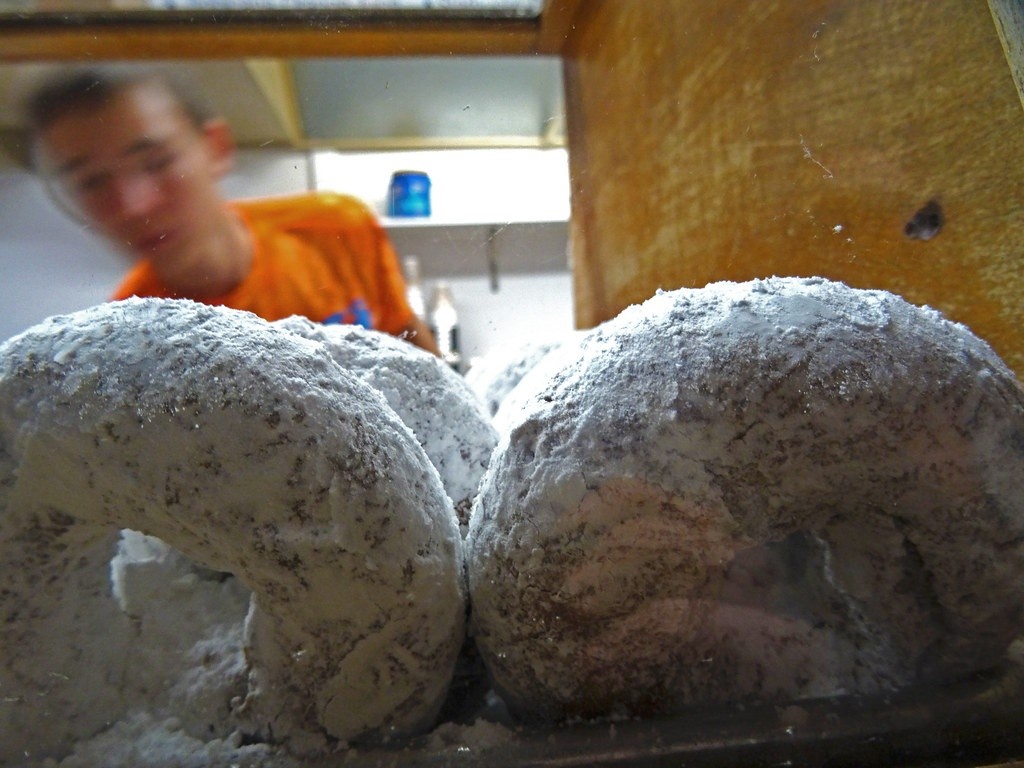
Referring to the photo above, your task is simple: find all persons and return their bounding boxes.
[18,58,443,360]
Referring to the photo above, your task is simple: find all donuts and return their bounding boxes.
[0,275,1024,768]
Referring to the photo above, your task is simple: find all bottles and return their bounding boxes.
[432,281,462,366]
[405,255,427,326]
